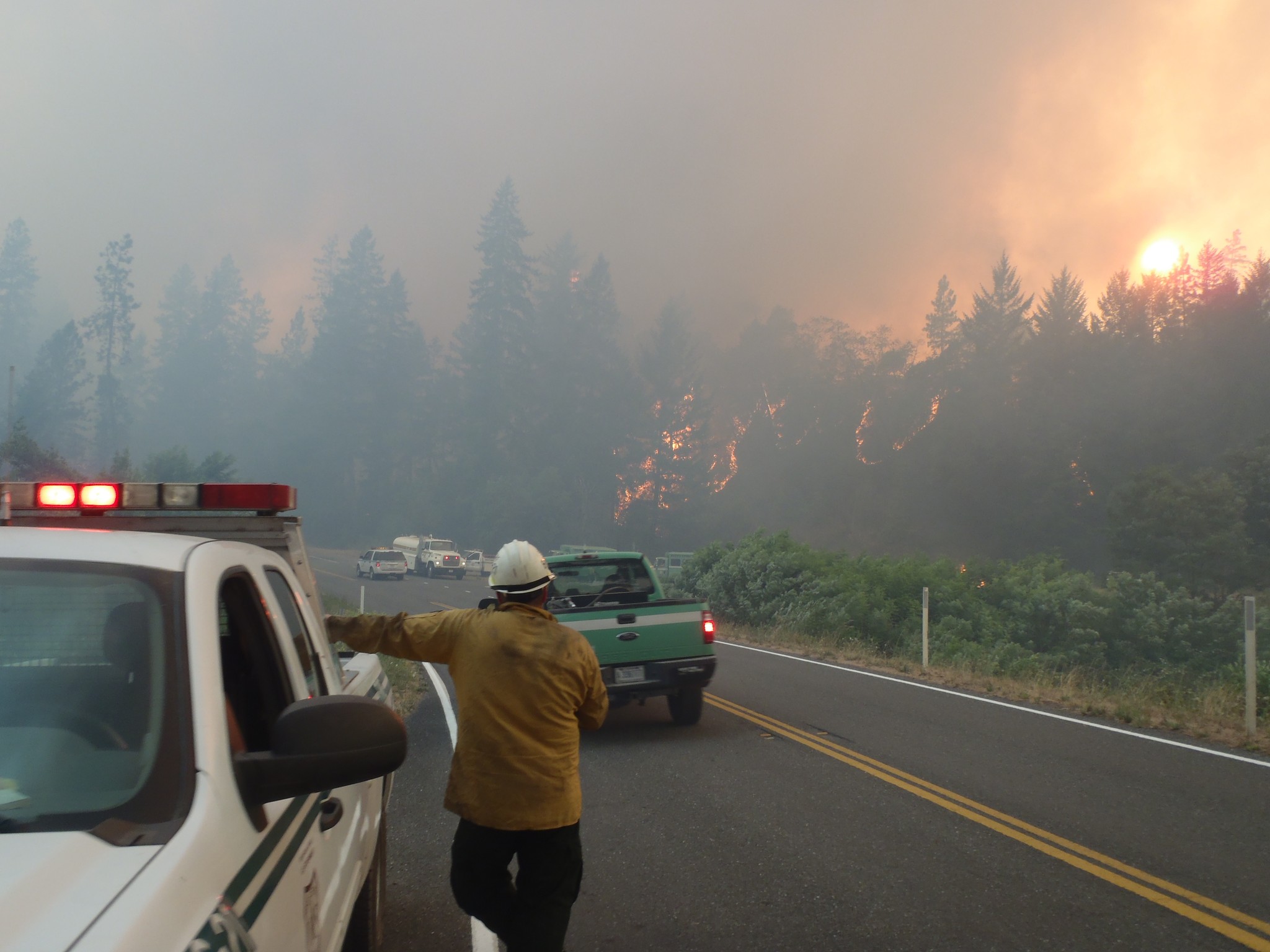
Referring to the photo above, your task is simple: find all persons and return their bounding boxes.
[324,540,609,952]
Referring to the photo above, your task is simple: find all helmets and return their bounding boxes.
[487,539,557,594]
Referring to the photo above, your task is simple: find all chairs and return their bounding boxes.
[600,574,626,594]
[103,603,148,733]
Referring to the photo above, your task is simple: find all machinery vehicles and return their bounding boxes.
[392,536,467,581]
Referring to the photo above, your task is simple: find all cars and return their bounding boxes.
[356,549,407,580]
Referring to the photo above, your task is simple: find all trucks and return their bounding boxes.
[654,551,694,584]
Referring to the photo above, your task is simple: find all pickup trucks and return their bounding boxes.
[479,551,716,725]
[461,549,496,577]
[0,524,410,952]
